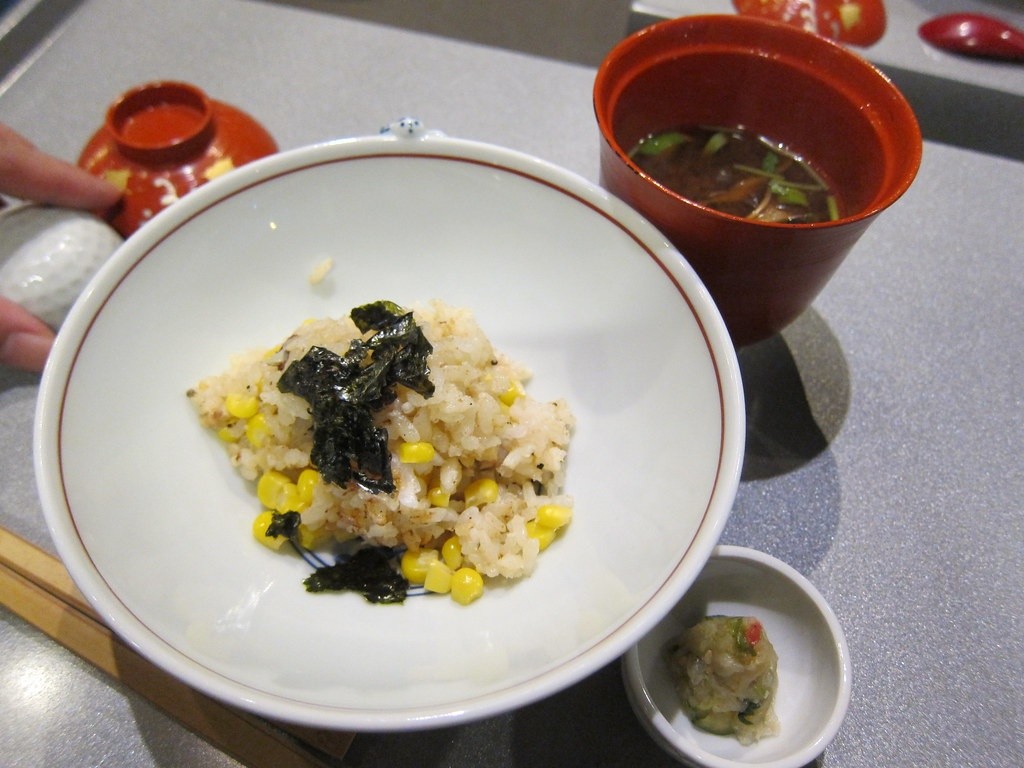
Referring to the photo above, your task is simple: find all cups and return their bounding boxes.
[593,15,924,350]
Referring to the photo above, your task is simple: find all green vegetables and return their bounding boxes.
[636,128,840,222]
[274,297,437,604]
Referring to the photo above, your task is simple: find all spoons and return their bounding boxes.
[915,14,1024,64]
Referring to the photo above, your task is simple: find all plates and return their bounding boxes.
[628,0,1024,96]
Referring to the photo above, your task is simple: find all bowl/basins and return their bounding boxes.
[621,545,852,768]
[31,114,746,732]
[77,80,279,242]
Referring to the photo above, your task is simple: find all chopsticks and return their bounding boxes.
[0,526,358,768]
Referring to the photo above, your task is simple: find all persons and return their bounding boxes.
[0,125,125,376]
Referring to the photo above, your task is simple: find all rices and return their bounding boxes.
[184,257,573,579]
